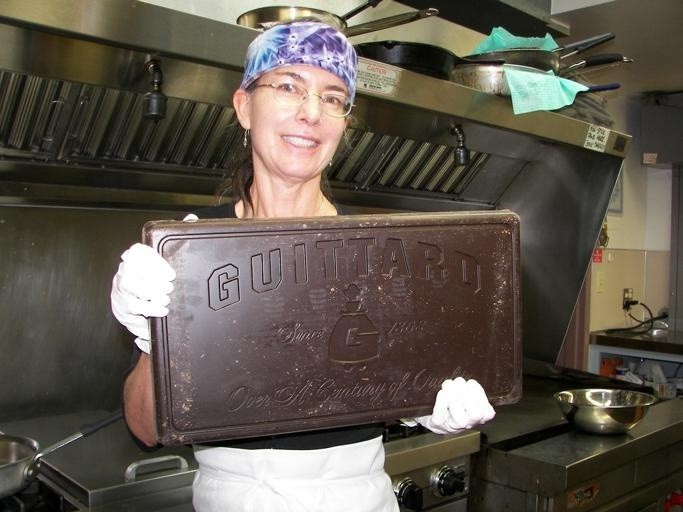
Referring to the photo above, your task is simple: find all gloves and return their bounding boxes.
[413,377,496,435]
[110,214,199,353]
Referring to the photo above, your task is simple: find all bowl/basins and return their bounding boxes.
[553,388,656,435]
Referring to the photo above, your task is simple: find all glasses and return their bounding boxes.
[256,82,357,118]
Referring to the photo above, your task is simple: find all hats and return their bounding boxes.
[242,21,358,106]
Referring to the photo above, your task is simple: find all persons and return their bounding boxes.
[112,27,497,511]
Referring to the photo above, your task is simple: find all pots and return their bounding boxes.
[238,0,629,96]
[0,410,127,496]
